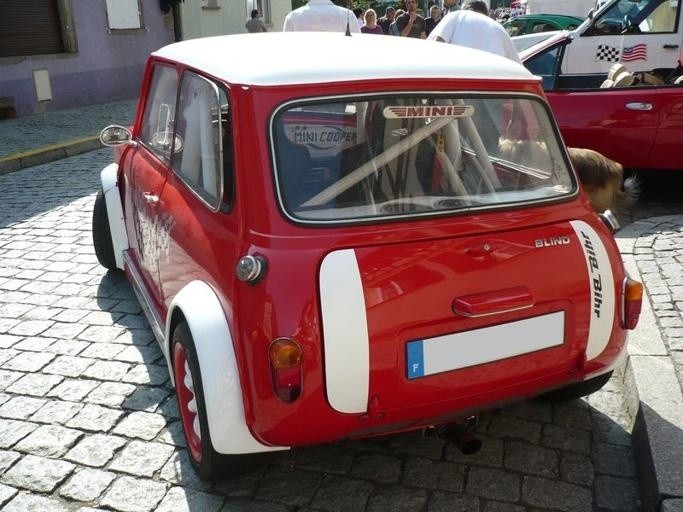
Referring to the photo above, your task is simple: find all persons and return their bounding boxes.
[282,0,520,66]
[245,10,267,33]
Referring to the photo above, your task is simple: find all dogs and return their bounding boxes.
[496,135,640,217]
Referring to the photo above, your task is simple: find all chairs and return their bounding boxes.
[337,98,447,206]
[597,63,634,89]
[197,102,311,205]
